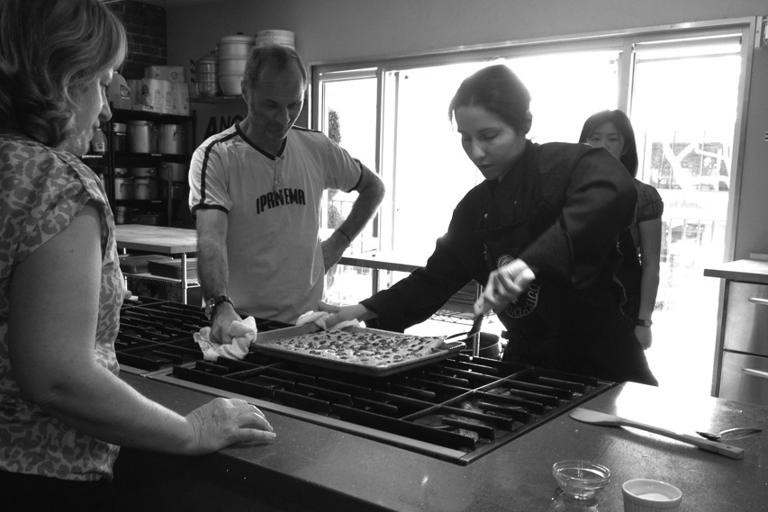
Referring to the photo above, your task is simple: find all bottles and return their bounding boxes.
[91,127,108,152]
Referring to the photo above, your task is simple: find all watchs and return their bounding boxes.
[636,318,652,327]
[202,295,231,321]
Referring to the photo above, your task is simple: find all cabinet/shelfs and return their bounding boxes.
[104,100,196,226]
[703,255,768,405]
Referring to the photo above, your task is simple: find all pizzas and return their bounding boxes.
[267,327,446,370]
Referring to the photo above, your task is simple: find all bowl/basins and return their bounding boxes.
[551,458,611,500]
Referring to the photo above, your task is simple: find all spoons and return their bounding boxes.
[696,426,763,440]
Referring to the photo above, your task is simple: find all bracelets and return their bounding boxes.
[334,226,352,243]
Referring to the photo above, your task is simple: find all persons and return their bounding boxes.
[317,63,657,386]
[577,108,666,353]
[2,0,278,512]
[185,43,386,348]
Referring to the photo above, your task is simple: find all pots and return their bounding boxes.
[189,30,296,97]
[113,118,187,224]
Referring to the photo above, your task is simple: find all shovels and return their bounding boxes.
[569,407,745,460]
[441,269,536,346]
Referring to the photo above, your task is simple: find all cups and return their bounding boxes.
[621,478,683,512]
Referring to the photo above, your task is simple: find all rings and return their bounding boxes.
[640,344,646,349]
